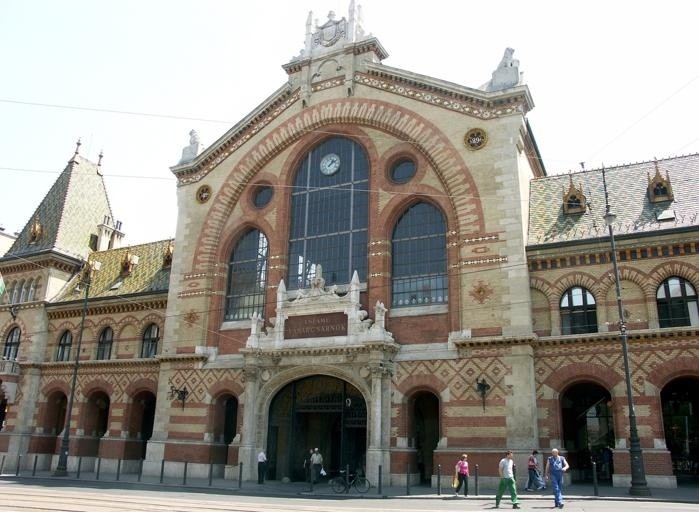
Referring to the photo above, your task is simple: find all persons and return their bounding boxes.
[303,448,323,484]
[526,450,545,491]
[257,449,267,484]
[496,450,521,509]
[455,454,469,497]
[545,448,569,509]
[416,446,424,473]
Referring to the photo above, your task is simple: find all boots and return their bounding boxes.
[513,504,521,509]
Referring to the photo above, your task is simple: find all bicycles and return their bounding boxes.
[331,469,371,494]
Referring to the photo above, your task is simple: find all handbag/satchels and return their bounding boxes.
[451,478,459,489]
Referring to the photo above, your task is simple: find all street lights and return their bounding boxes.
[52,257,92,476]
[601,169,651,496]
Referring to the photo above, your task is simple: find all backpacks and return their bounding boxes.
[533,470,547,490]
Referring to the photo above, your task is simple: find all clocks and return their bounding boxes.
[320,153,340,175]
